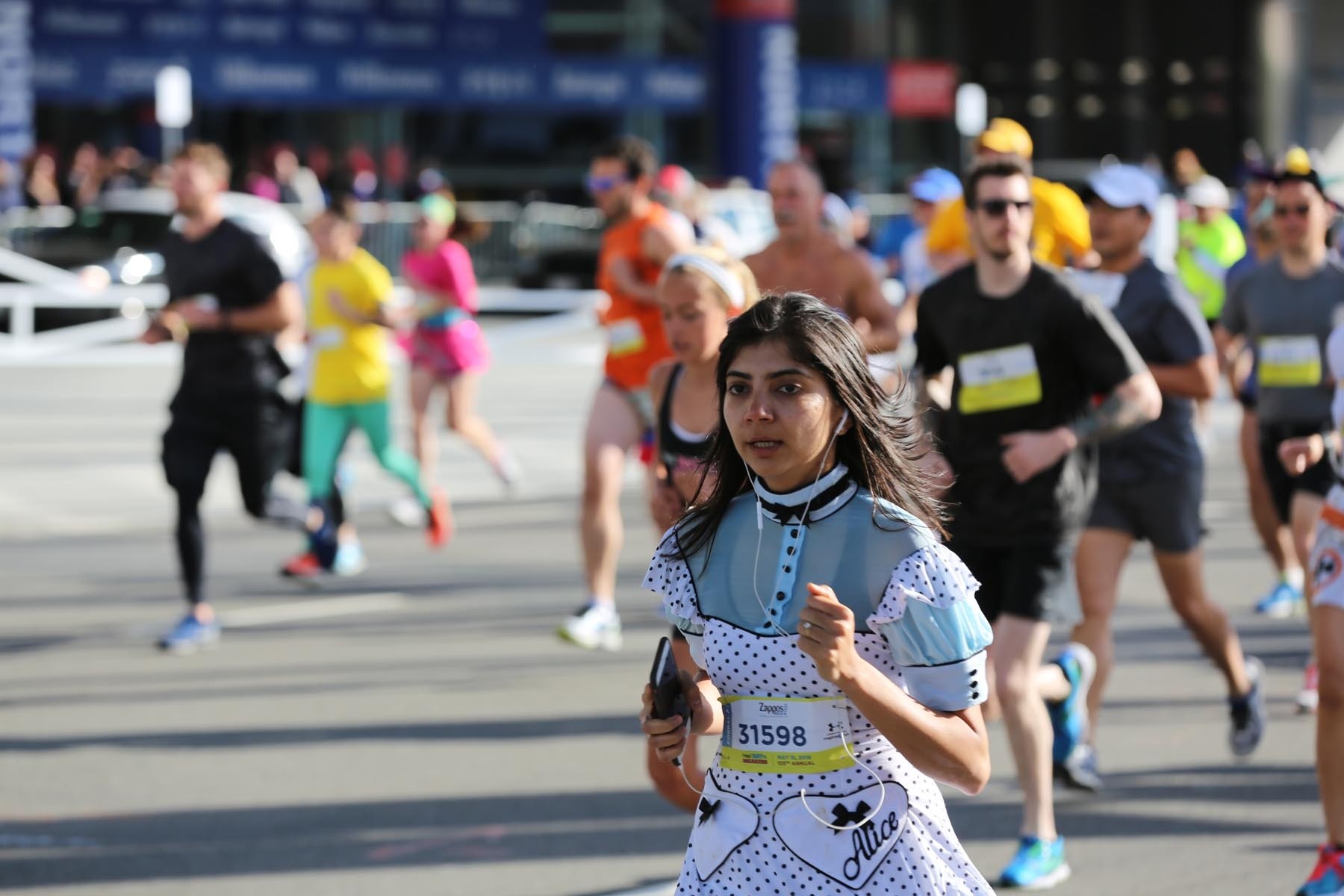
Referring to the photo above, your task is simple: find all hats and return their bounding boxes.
[1077,163,1160,218]
[910,167,963,205]
[978,117,1033,160]
[1184,174,1228,209]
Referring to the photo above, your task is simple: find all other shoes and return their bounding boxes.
[333,539,369,576]
[557,604,623,651]
[425,488,453,543]
[156,616,220,653]
[284,551,329,578]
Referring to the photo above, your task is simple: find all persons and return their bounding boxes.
[1062,171,1264,790]
[0,141,519,661]
[900,117,1246,430]
[548,139,900,654]
[913,162,1161,891]
[1219,142,1344,896]
[640,295,999,896]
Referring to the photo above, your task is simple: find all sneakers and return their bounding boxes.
[1229,657,1267,752]
[1293,660,1320,712]
[999,832,1070,888]
[1294,840,1344,896]
[1043,642,1097,763]
[1256,582,1310,619]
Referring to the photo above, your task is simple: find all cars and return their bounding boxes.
[0,183,320,358]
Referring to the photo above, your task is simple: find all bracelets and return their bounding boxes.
[1069,415,1099,448]
[1318,430,1333,453]
[219,309,231,329]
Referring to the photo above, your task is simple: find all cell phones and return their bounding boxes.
[649,636,694,767]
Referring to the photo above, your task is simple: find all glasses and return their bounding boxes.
[587,173,630,191]
[972,198,1033,216]
[1270,204,1327,219]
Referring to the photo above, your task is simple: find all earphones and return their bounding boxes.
[833,406,850,437]
